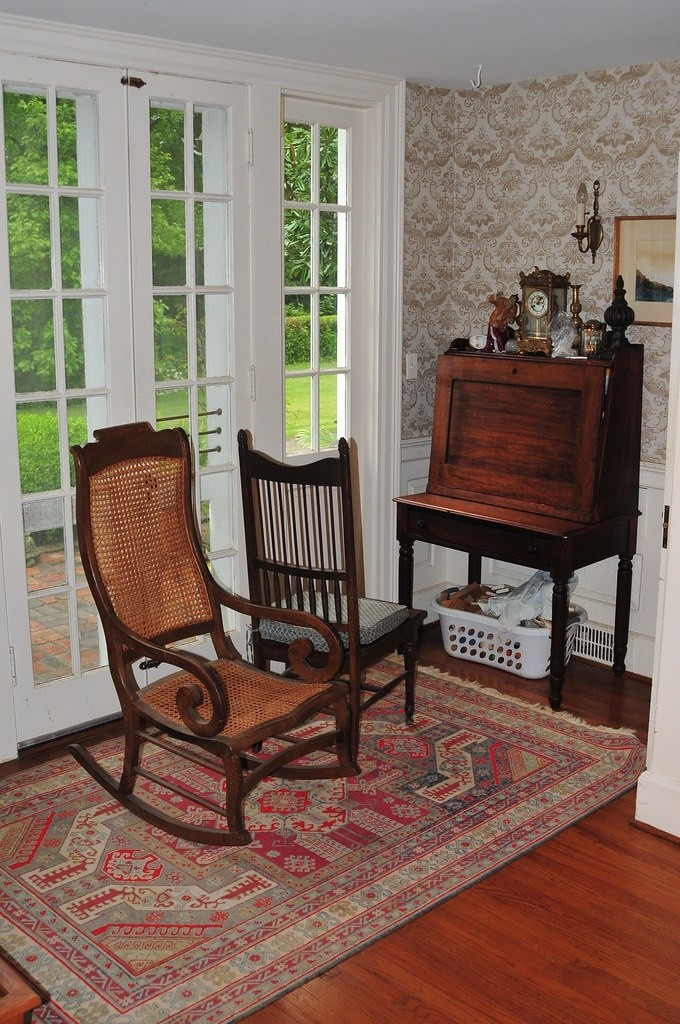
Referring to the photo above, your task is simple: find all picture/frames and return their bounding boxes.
[612,214,676,329]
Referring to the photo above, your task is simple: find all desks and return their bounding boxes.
[388,493,642,710]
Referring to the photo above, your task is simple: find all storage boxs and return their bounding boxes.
[431,588,588,679]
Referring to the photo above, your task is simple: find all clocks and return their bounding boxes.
[517,266,571,355]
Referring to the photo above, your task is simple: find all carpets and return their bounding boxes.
[0,650,648,1024]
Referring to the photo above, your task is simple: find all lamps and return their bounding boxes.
[567,180,602,265]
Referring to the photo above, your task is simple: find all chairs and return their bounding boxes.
[231,427,429,757]
[66,422,361,849]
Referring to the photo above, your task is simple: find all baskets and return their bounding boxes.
[431,584,587,678]
